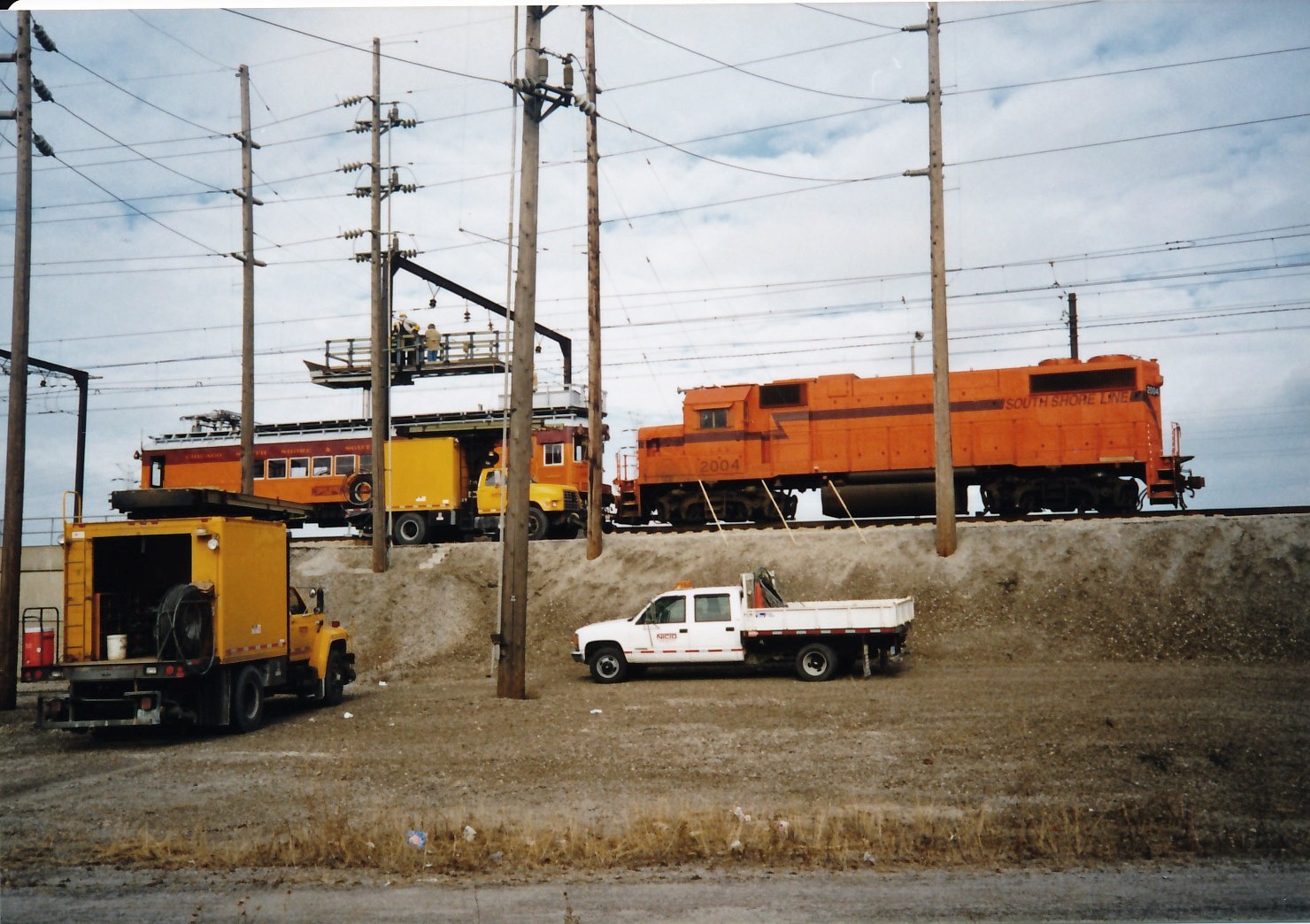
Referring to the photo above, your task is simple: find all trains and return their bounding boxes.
[613,352,1205,531]
[133,402,611,541]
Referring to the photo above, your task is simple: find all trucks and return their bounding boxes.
[380,434,582,544]
[19,485,358,734]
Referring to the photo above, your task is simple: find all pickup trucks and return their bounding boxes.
[573,571,916,682]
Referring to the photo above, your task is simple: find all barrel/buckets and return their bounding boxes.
[25,627,54,679]
[107,634,128,661]
